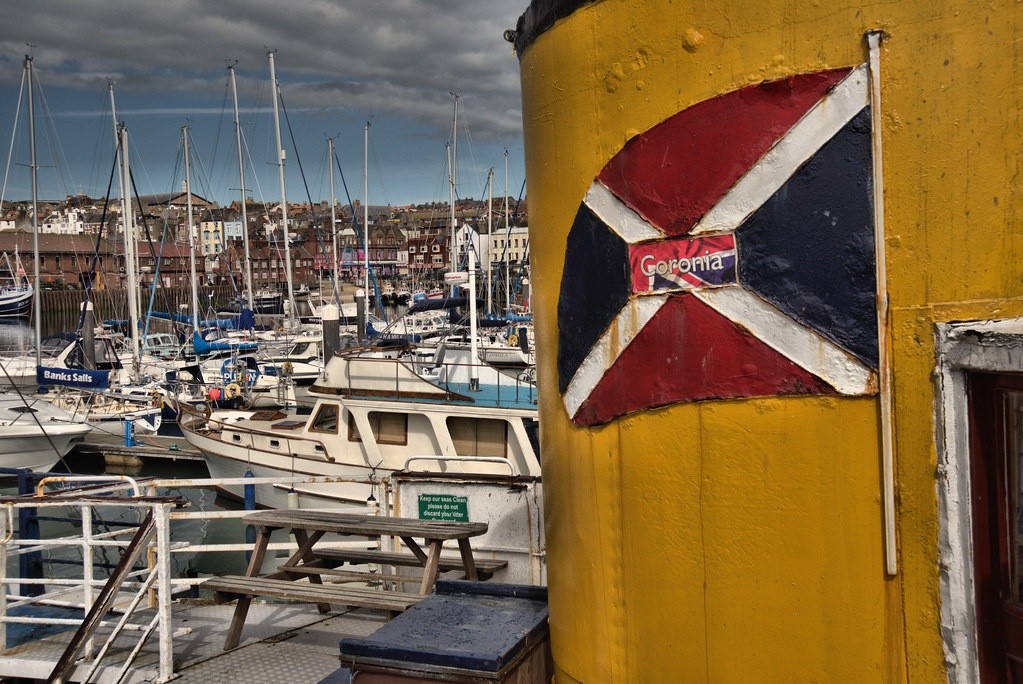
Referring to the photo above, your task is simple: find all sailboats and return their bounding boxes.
[0,53,541,435]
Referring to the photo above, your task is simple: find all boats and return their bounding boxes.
[173,247,541,516]
[1,391,92,481]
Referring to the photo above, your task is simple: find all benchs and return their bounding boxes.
[200,574,430,611]
[311,548,510,573]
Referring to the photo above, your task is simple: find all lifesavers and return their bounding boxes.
[225,383,240,398]
[506,335,518,346]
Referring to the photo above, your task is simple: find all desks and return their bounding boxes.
[224,510,493,650]
